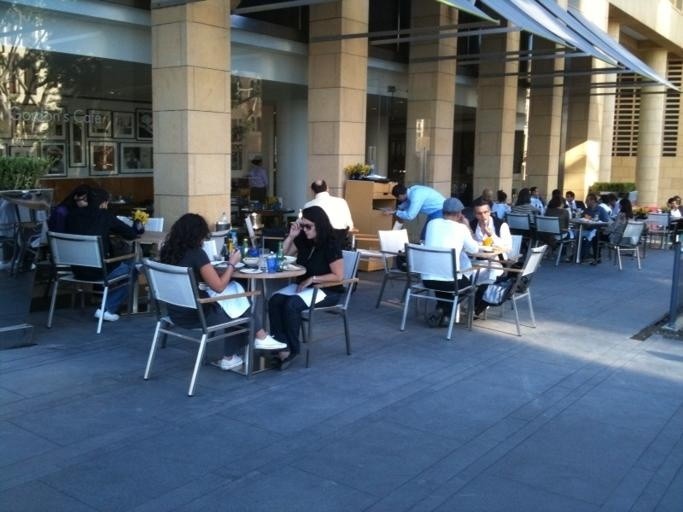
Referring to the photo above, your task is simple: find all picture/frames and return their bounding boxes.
[0,102,153,177]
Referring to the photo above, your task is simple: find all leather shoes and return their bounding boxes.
[281,352,299,370]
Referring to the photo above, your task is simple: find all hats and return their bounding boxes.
[443,197,465,212]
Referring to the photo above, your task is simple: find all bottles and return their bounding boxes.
[219,232,284,272]
[220,212,227,224]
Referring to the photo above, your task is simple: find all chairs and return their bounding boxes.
[376,228,420,308]
[400,243,480,340]
[491,206,683,271]
[467,244,548,336]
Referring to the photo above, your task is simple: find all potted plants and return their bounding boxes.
[345,162,370,179]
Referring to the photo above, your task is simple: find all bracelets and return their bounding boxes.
[229,264,236,272]
[313,275,317,284]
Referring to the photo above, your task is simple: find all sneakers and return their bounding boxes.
[221,354,243,370]
[254,335,287,349]
[95,308,120,321]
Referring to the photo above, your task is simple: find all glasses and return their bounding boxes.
[299,224,314,229]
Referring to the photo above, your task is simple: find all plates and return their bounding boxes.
[240,269,263,274]
[210,261,245,268]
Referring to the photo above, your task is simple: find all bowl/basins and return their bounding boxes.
[268,255,298,264]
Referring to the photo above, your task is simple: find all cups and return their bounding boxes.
[483,237,492,246]
[595,215,599,222]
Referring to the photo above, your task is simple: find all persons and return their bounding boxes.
[268,206,346,370]
[469,198,513,321]
[385,183,444,245]
[248,155,268,203]
[65,190,138,322]
[159,213,287,372]
[472,184,683,264]
[426,198,479,327]
[47,184,95,302]
[310,180,354,249]
[231,178,252,226]
